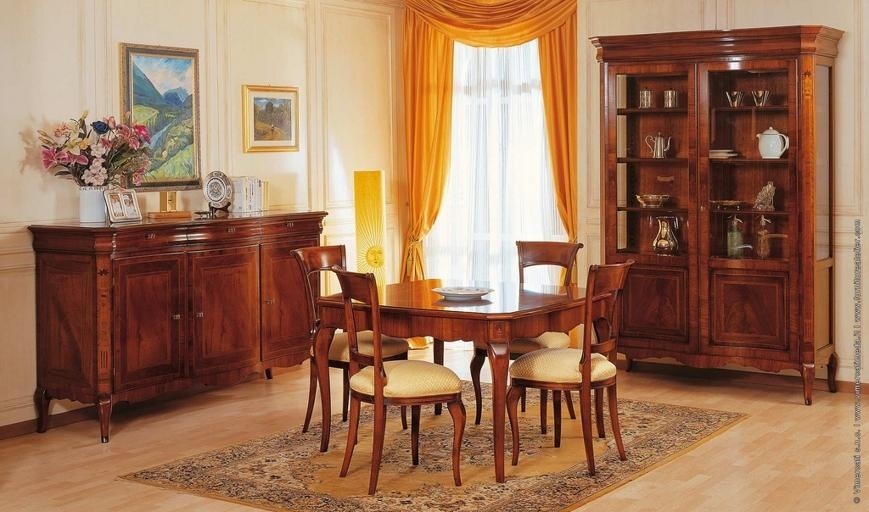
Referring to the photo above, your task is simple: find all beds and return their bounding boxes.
[306,274,615,484]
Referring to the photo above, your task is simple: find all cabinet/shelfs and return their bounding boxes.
[582,22,848,408]
[23,213,262,445]
[255,208,333,383]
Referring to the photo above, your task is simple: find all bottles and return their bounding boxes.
[756,215,773,257]
[727,218,745,259]
[652,216,679,256]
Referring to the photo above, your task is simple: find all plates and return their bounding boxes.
[202,171,233,209]
[709,149,738,159]
[432,287,493,302]
[710,200,748,207]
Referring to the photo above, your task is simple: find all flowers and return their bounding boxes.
[32,103,155,193]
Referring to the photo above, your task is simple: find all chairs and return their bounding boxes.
[464,234,585,424]
[285,239,412,456]
[325,259,466,498]
[497,255,644,480]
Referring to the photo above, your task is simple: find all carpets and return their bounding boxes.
[117,377,752,512]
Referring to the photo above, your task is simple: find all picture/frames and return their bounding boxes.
[101,184,144,225]
[113,38,209,195]
[239,81,301,153]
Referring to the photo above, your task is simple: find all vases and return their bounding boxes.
[73,181,115,225]
[652,214,681,258]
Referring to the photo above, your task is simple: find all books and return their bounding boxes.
[229,175,271,213]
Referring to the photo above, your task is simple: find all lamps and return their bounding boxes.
[352,166,389,307]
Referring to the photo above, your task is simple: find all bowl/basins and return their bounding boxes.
[635,195,670,209]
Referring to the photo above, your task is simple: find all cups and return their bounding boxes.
[663,89,678,109]
[725,91,744,108]
[639,89,656,109]
[752,90,770,107]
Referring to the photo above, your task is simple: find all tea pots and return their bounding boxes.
[756,127,789,160]
[645,132,672,159]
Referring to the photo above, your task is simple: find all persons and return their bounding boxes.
[122,195,138,217]
[110,197,123,217]
